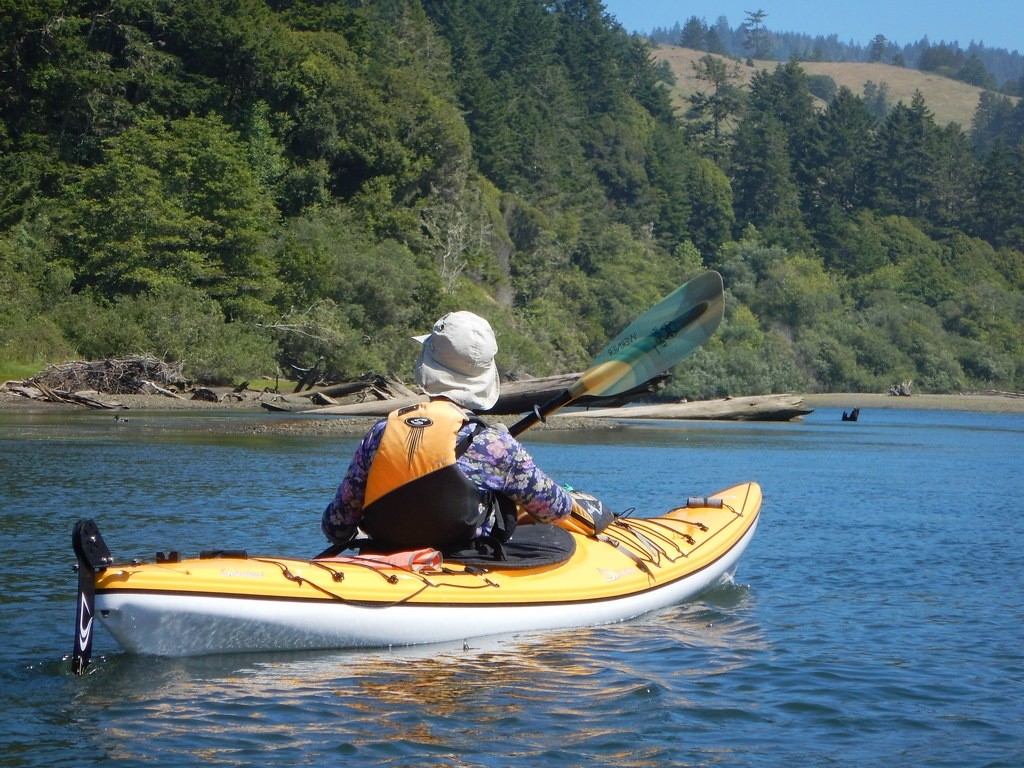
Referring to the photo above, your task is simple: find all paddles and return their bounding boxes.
[308,261,732,566]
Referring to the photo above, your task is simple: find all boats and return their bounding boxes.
[71,481,763,677]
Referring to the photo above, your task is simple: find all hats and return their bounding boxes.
[415,311,500,411]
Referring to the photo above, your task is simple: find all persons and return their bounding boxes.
[322,310,610,551]
